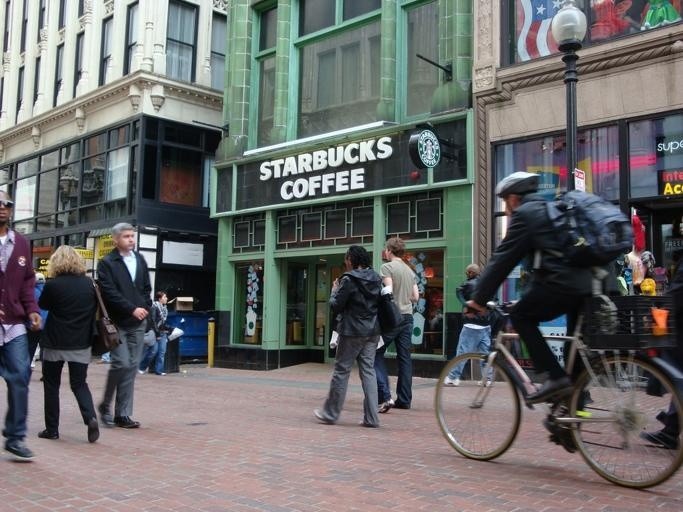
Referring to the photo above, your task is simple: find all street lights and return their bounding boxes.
[547,0,588,333]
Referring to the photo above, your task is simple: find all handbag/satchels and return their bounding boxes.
[379,294,403,332]
[89,277,122,357]
[142,329,156,346]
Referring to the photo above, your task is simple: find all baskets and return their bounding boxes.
[583,291,680,352]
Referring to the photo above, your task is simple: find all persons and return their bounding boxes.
[313,244,386,429]
[373,237,417,410]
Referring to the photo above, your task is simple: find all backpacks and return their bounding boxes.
[521,189,634,270]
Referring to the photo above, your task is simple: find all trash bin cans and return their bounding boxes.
[149,325,181,373]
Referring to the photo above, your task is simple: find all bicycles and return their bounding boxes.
[432,285,682,492]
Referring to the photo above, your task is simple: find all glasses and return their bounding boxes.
[0,200,14,209]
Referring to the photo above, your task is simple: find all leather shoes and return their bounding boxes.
[523,375,575,405]
[99,403,114,425]
[640,429,680,451]
[114,416,141,428]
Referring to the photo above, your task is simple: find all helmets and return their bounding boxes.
[495,170,540,199]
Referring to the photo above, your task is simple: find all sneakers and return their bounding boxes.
[478,379,495,388]
[37,428,59,439]
[443,376,460,387]
[313,409,333,425]
[3,440,34,461]
[379,400,412,414]
[88,416,100,443]
